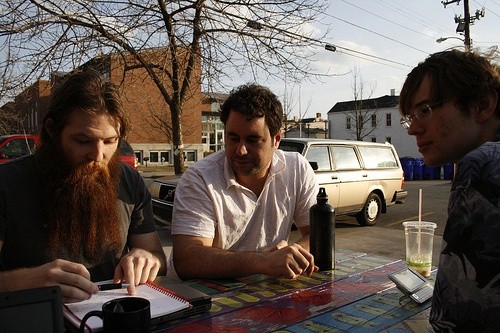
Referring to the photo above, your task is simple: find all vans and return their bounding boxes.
[0,134,140,174]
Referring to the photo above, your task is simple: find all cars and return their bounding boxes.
[153,137,411,241]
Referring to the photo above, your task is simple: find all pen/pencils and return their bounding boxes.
[98,283,130,291]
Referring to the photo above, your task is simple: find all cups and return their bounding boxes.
[79,297,151,333]
[402,221,437,277]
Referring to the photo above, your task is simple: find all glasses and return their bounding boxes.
[400,103,445,130]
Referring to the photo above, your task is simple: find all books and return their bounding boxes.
[61,278,193,333]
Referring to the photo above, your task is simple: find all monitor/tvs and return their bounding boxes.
[389,265,429,295]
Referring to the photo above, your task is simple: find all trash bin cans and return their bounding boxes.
[399,156,453,181]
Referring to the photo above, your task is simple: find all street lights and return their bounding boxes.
[436,35,472,55]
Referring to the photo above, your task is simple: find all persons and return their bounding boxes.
[0,72,168,305]
[399,49,500,333]
[170,84,320,282]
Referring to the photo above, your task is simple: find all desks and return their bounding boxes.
[152,249,439,333]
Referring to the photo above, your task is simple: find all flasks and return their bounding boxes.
[309,187,335,271]
